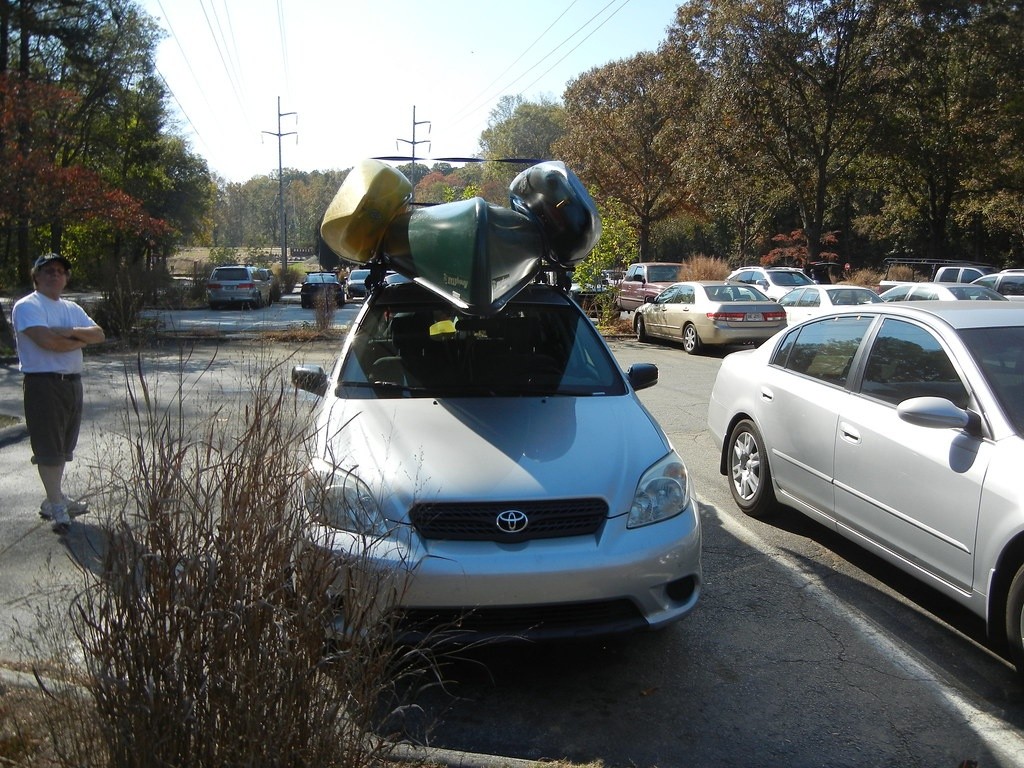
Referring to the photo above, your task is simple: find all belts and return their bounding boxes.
[37,372,82,381]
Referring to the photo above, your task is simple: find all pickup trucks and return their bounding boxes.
[877,266,999,293]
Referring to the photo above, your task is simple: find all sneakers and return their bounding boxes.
[51,503,71,533]
[37,494,87,517]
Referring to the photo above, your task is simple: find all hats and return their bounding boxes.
[34,251,72,270]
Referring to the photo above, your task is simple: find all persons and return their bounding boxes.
[12,253,106,529]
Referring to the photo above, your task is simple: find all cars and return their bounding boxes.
[707,300,1024,691]
[612,262,690,311]
[772,284,886,327]
[300,272,345,309]
[207,264,275,309]
[631,280,788,356]
[861,282,1011,301]
[563,268,627,318]
[344,270,372,299]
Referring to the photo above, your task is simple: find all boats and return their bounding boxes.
[319,155,413,261]
[510,160,601,269]
[390,194,546,316]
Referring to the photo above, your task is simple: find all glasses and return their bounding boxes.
[42,267,68,276]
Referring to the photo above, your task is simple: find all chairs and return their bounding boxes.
[369,312,561,374]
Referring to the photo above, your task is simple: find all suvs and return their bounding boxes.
[290,267,704,648]
[714,265,819,304]
[964,269,1024,302]
